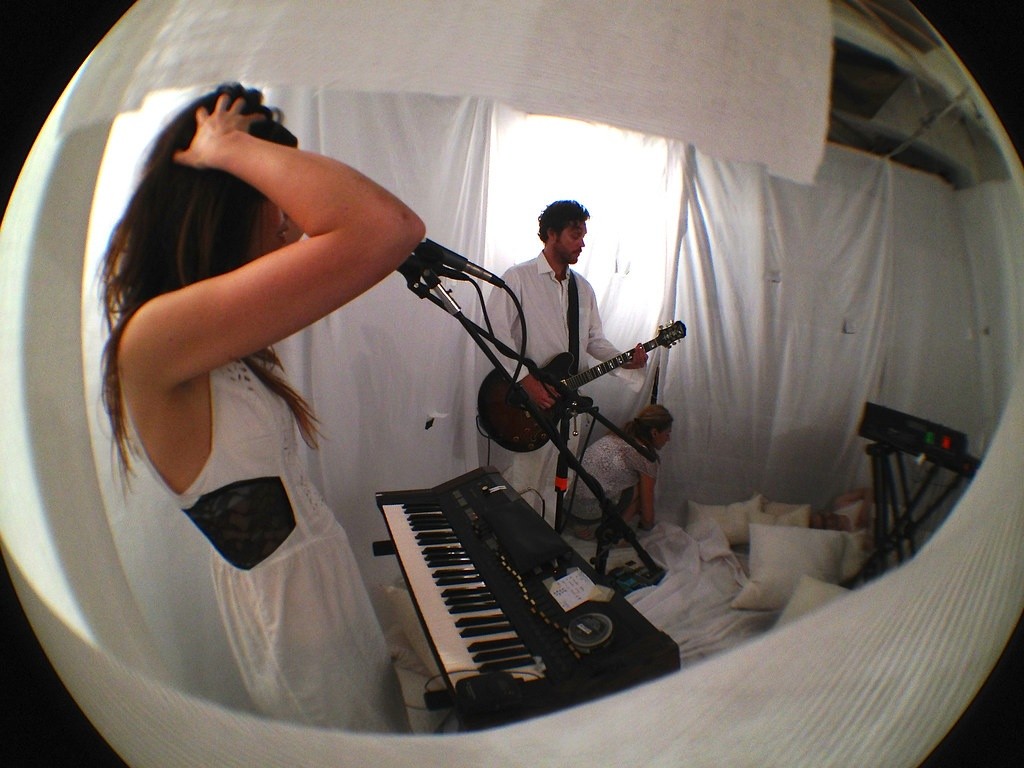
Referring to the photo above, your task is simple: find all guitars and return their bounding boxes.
[475,314,686,452]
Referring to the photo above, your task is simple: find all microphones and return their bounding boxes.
[414,237,505,289]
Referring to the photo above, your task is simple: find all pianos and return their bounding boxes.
[373,465,686,736]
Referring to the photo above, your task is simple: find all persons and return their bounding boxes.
[481,200,648,531]
[807,486,908,560]
[561,405,674,547]
[103,82,426,735]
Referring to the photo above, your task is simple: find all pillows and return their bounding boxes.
[748,503,811,528]
[769,576,854,634]
[728,523,844,611]
[687,494,763,544]
